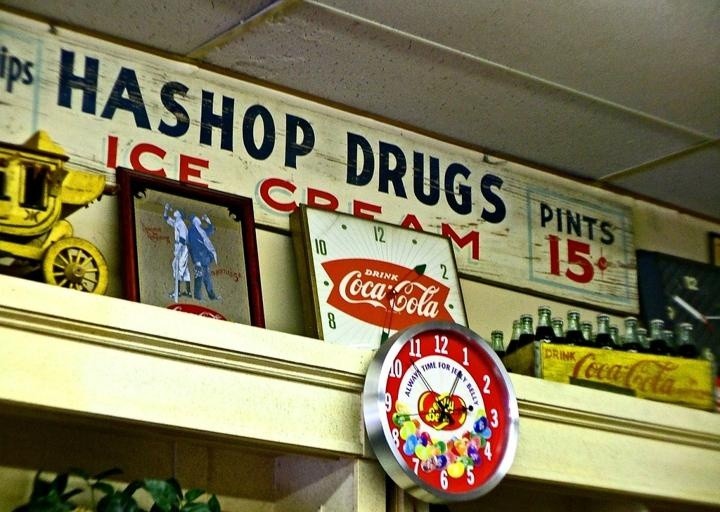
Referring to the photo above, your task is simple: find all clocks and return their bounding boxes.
[639,245,718,356]
[365,322,521,503]
[289,198,477,356]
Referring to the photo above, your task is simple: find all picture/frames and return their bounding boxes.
[115,164,270,332]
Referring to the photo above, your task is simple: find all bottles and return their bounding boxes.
[490,308,700,360]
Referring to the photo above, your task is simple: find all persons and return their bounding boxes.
[164,203,190,298]
[187,213,218,299]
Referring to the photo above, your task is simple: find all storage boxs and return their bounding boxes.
[500,337,717,412]
